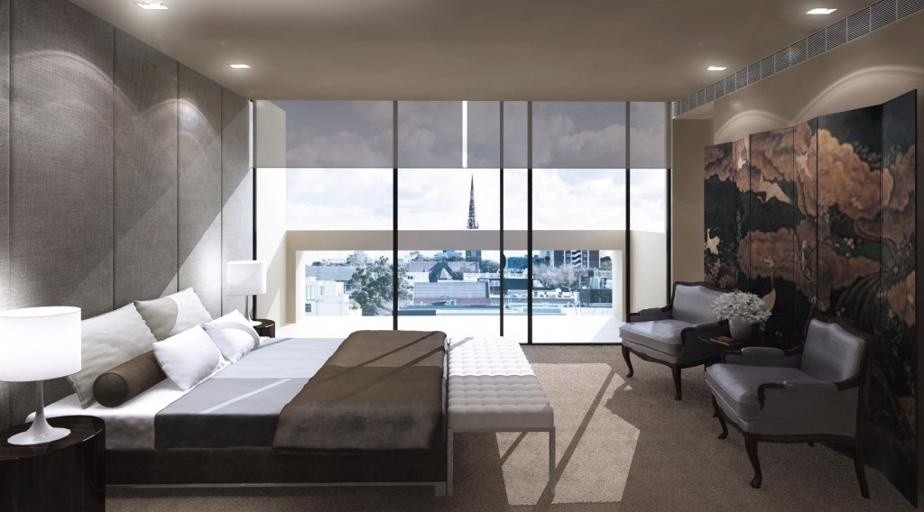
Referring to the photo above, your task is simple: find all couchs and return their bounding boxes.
[702,309,873,499]
[619,280,735,401]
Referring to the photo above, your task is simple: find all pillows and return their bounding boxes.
[153,323,221,391]
[204,307,261,365]
[135,287,214,341]
[65,302,157,410]
[93,351,167,408]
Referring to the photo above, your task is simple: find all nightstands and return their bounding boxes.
[0,416,106,512]
[250,318,275,338]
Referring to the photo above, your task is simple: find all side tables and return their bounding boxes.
[698,322,805,418]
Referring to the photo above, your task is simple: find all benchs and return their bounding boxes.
[447,333,556,499]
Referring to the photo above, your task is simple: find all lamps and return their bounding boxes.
[224,259,268,321]
[0,305,83,447]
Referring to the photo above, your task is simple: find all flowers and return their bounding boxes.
[710,284,773,332]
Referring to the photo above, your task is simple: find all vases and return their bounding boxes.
[728,316,759,341]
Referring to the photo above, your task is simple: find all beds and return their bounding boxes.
[9,331,447,498]
